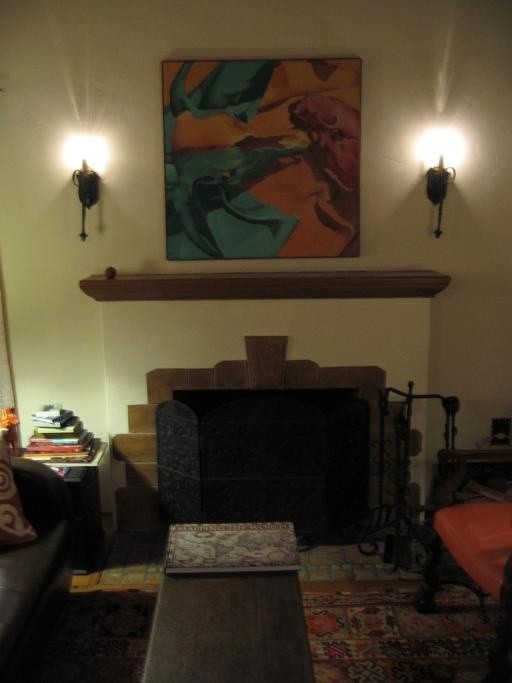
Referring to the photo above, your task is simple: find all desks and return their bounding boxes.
[143,520,318,683]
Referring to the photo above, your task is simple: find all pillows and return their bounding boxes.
[1,446,39,547]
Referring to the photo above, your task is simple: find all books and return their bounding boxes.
[163,520,301,574]
[21,403,108,468]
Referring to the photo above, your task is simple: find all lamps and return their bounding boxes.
[57,127,113,242]
[411,124,470,241]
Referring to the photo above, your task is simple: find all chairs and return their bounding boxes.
[419,499,511,621]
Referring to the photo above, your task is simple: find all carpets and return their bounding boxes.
[16,565,510,680]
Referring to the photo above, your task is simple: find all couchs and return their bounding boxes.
[2,453,86,682]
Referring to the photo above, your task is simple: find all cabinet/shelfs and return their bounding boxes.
[21,440,117,573]
